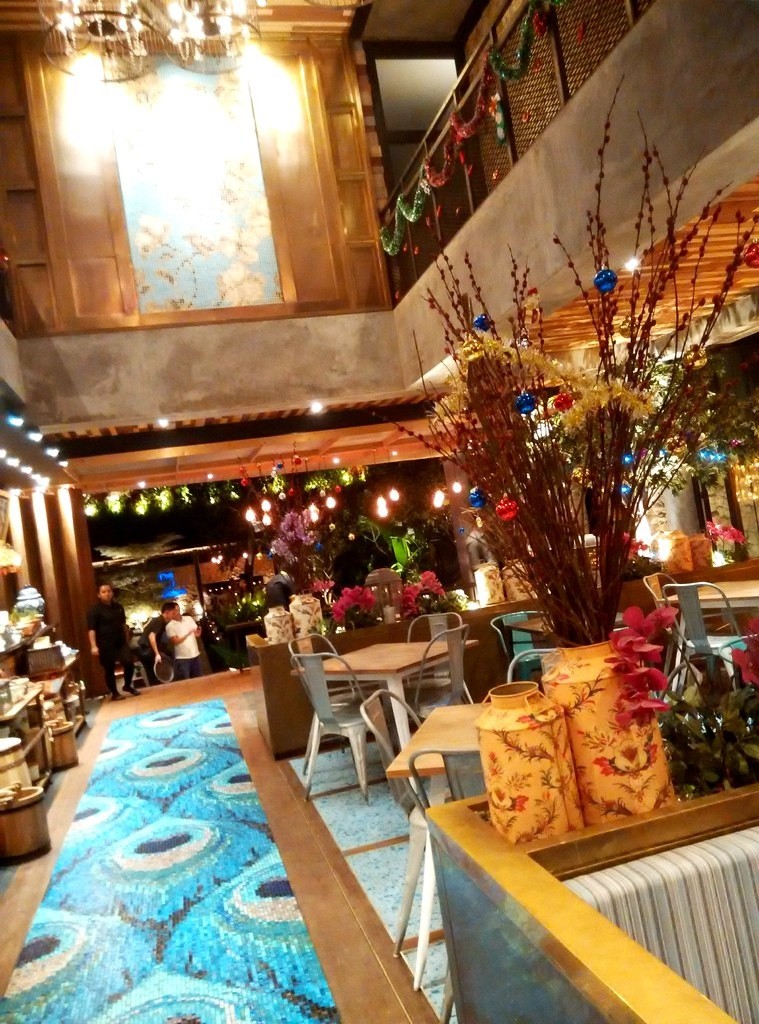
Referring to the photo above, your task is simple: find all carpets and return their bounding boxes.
[0,695,342,1024]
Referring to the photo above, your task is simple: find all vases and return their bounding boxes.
[473,562,505,606]
[501,559,535,601]
[288,593,324,638]
[344,604,369,630]
[689,529,713,570]
[540,641,678,827]
[416,589,440,615]
[657,529,694,574]
[472,682,585,845]
[263,606,295,644]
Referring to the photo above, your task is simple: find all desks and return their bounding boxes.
[656,580,759,692]
[384,702,491,993]
[290,640,479,796]
[503,612,624,637]
[225,620,263,674]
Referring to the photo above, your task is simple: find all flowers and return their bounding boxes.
[705,521,749,564]
[230,439,342,595]
[602,605,759,797]
[622,533,649,571]
[400,570,446,618]
[331,586,376,625]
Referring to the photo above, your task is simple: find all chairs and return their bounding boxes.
[643,571,731,678]
[718,634,756,665]
[661,582,743,693]
[409,748,486,1024]
[359,688,457,958]
[405,614,475,720]
[289,633,376,776]
[489,611,549,681]
[290,651,387,805]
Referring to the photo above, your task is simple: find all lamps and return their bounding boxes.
[157,0,267,75]
[37,0,168,84]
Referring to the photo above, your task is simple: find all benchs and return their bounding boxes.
[550,810,759,1024]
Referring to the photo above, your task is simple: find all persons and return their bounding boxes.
[264,564,296,612]
[86,582,141,700]
[136,602,176,686]
[165,603,203,679]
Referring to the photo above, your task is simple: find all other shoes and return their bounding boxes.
[112,693,125,700]
[122,685,141,695]
